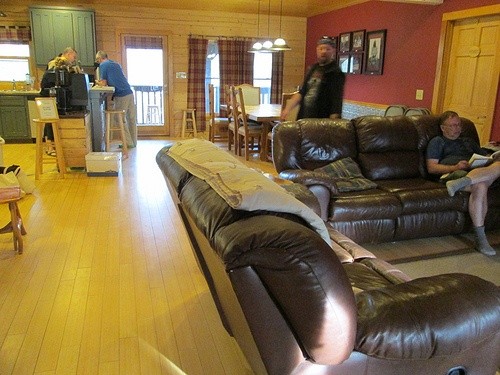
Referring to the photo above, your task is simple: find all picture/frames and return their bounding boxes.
[322,28,365,75]
[362,28,387,76]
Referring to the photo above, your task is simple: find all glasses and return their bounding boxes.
[443,124,462,129]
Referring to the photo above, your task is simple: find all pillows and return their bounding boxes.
[315,157,378,194]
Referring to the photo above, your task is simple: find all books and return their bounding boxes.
[468,150,500,168]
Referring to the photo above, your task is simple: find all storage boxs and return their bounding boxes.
[84,152,121,176]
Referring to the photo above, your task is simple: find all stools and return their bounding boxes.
[104,109,129,158]
[32,118,66,180]
[182,109,198,138]
[0,187,26,254]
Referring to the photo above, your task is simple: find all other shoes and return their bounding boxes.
[45,151,57,157]
[118,144,136,148]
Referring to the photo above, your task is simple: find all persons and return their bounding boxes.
[280,39,345,121]
[96,50,137,147]
[40,47,76,156]
[428,111,500,255]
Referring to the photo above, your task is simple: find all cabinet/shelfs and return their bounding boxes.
[0,95,31,141]
[58,112,93,171]
[29,5,98,68]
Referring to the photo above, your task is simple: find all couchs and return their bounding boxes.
[272,113,500,245]
[155,139,499,375]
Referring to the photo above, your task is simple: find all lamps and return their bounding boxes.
[247,0,294,54]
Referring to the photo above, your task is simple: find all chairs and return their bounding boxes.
[384,104,433,117]
[209,83,302,161]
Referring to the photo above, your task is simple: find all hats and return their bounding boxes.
[316,38,336,48]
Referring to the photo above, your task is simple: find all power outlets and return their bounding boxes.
[416,89,424,100]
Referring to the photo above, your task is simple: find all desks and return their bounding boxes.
[219,96,282,161]
